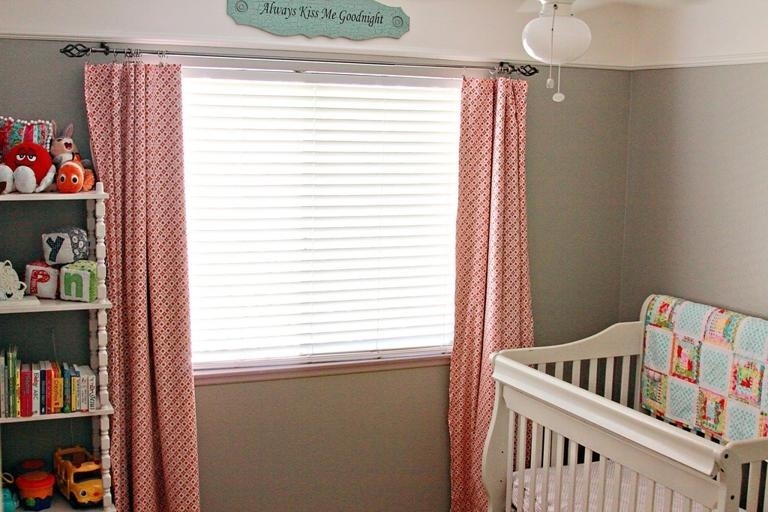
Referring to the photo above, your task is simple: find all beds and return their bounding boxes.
[480,295,768,512]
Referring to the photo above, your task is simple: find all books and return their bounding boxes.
[0,344,97,419]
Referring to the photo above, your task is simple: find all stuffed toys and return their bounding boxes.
[0,115,96,195]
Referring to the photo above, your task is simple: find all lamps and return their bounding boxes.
[521,0,592,103]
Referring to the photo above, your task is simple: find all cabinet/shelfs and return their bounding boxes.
[0,182,115,511]
